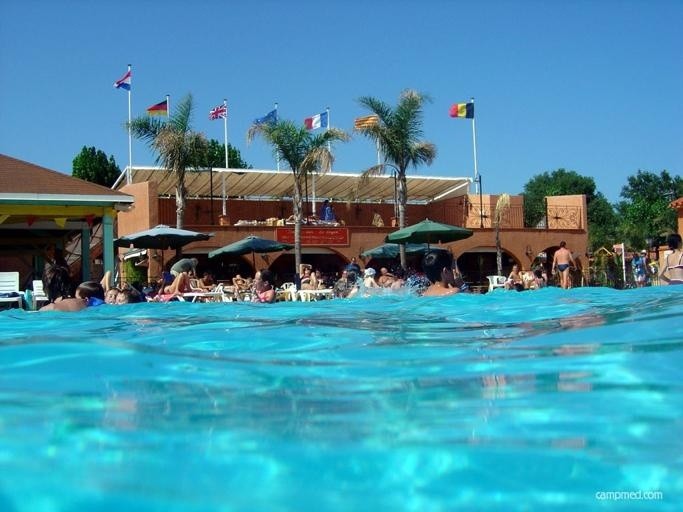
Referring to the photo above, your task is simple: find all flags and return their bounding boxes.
[252,109,279,127]
[449,103,475,118]
[354,114,379,129]
[147,99,168,117]
[113,71,132,91]
[209,104,227,120]
[304,111,328,132]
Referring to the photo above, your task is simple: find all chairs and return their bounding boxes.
[31,280,49,310]
[0,271,22,308]
[144,274,333,303]
[487,276,506,292]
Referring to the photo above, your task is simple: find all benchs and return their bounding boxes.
[505,271,547,290]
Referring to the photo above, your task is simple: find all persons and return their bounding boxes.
[551,241,577,289]
[35,249,549,313]
[631,233,683,288]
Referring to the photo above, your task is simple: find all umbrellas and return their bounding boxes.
[113,224,216,273]
[208,233,295,269]
[383,218,473,253]
[360,241,447,258]
